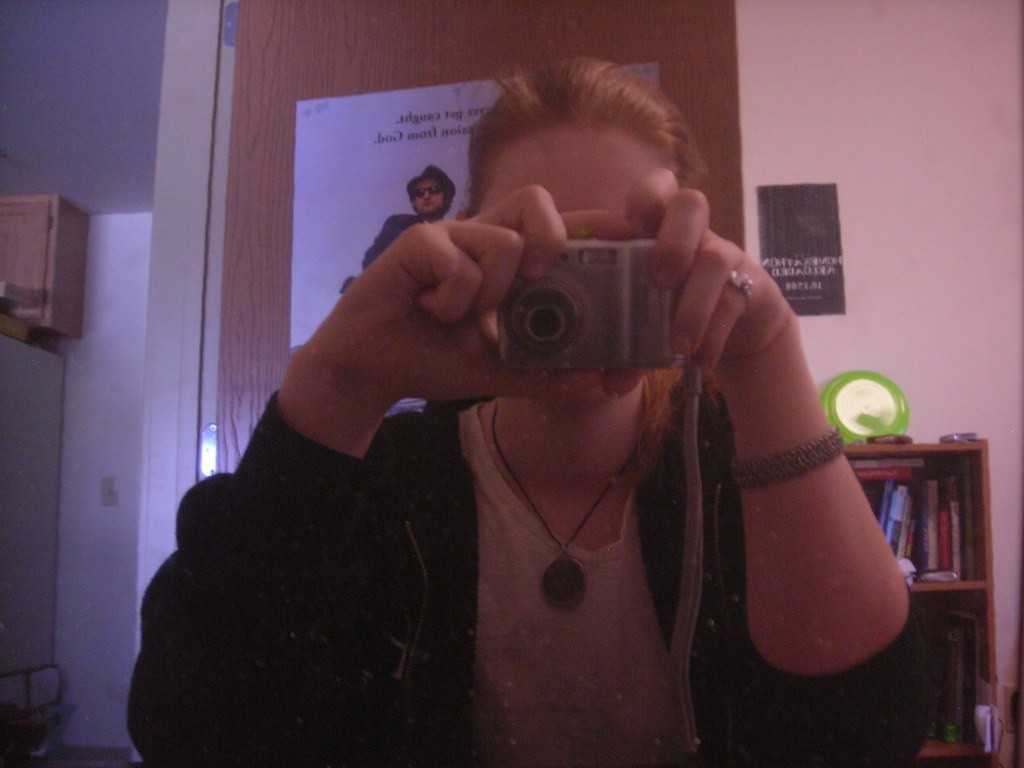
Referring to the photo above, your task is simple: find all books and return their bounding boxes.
[879,475,980,744]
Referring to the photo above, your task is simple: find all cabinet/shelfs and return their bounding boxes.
[0,195,89,340]
[0,335,66,707]
[843,438,999,768]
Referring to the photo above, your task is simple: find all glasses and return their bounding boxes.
[412,185,443,197]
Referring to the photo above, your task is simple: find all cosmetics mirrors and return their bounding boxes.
[822,369,910,444]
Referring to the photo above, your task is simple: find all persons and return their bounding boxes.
[362,165,456,271]
[126,57,931,768]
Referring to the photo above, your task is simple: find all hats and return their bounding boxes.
[407,165,456,200]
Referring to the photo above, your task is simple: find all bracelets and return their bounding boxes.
[734,429,847,489]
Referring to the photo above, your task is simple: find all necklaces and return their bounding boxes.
[492,402,637,606]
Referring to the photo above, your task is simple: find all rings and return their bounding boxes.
[727,273,753,301]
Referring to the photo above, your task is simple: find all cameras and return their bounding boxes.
[497,238,673,370]
